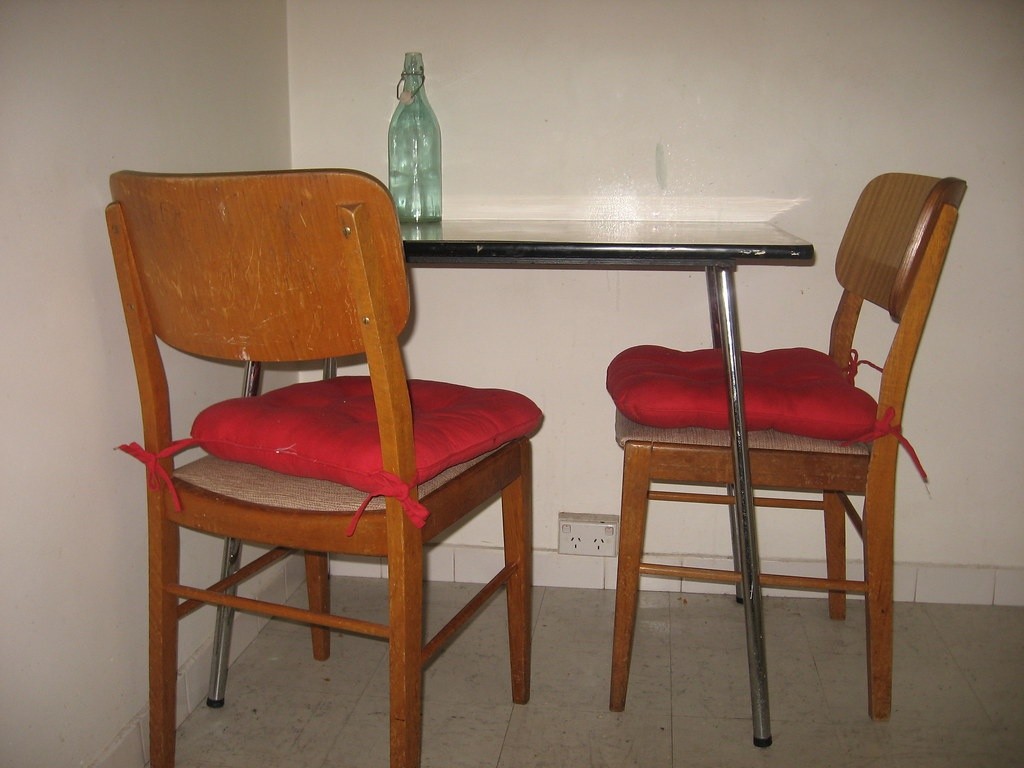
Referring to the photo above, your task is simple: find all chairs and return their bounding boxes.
[105,167,546,768]
[607,170,969,712]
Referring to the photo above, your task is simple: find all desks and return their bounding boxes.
[205,218,816,750]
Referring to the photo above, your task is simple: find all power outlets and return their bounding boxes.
[558,512,619,558]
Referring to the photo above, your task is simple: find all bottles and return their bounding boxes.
[388,54,441,225]
